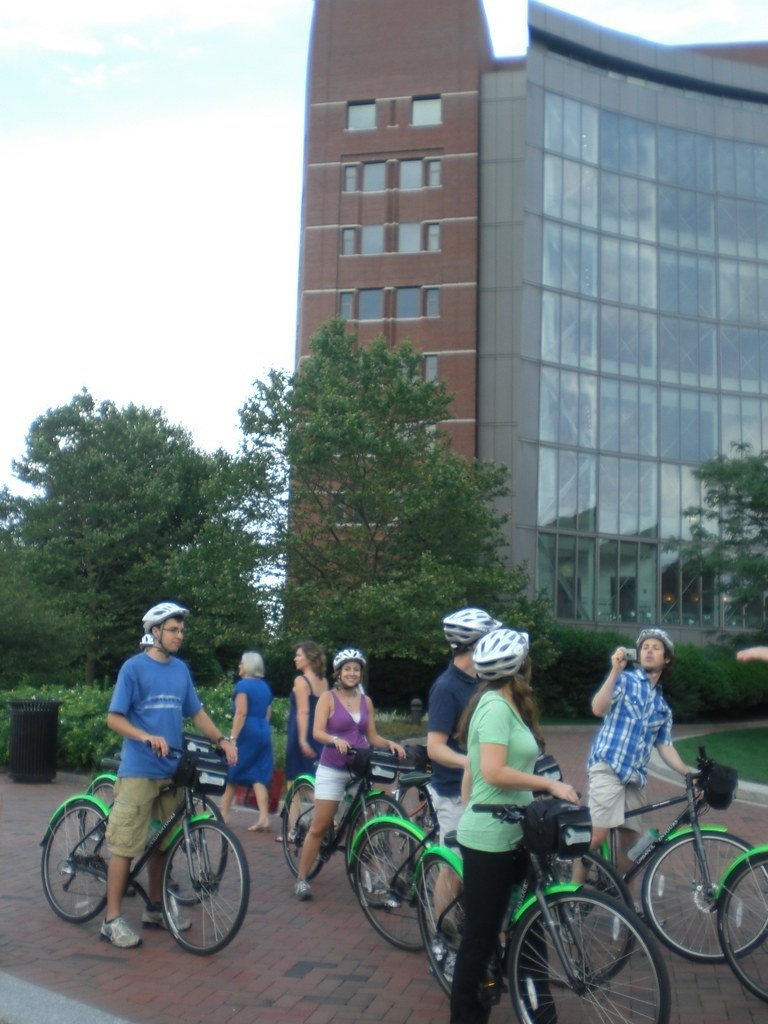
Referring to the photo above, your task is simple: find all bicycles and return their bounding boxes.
[382,740,768,965]
[278,740,417,910]
[76,729,228,906]
[345,752,639,994]
[708,845,768,1007]
[38,736,251,957]
[408,801,674,1024]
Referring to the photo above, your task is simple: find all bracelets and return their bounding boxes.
[229,736,235,740]
[330,736,338,743]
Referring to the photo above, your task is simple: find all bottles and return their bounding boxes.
[138,819,163,854]
[333,794,353,825]
[502,885,522,933]
[627,828,660,864]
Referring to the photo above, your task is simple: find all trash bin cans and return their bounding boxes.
[8,699,64,783]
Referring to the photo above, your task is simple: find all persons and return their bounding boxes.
[571,629,702,929]
[291,647,408,901]
[737,646,768,662]
[425,607,503,985]
[98,601,238,949]
[272,640,329,844]
[446,626,583,1024]
[220,650,274,834]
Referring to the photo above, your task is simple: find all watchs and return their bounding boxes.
[218,736,230,745]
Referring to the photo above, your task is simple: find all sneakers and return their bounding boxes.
[363,876,387,894]
[99,915,142,948]
[140,908,193,932]
[295,878,312,899]
[430,941,457,980]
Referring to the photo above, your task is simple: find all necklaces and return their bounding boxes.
[346,703,354,714]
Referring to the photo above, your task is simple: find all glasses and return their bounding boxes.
[160,627,184,634]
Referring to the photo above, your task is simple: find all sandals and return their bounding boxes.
[274,832,298,844]
[247,818,271,833]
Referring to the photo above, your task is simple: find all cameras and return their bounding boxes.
[625,649,637,661]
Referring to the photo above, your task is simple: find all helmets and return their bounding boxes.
[472,628,529,680]
[637,627,675,660]
[332,650,367,672]
[140,634,154,649]
[142,603,190,634]
[442,607,504,645]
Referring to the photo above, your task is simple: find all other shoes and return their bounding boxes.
[168,877,179,892]
[125,886,137,897]
[561,904,581,944]
[636,911,665,929]
